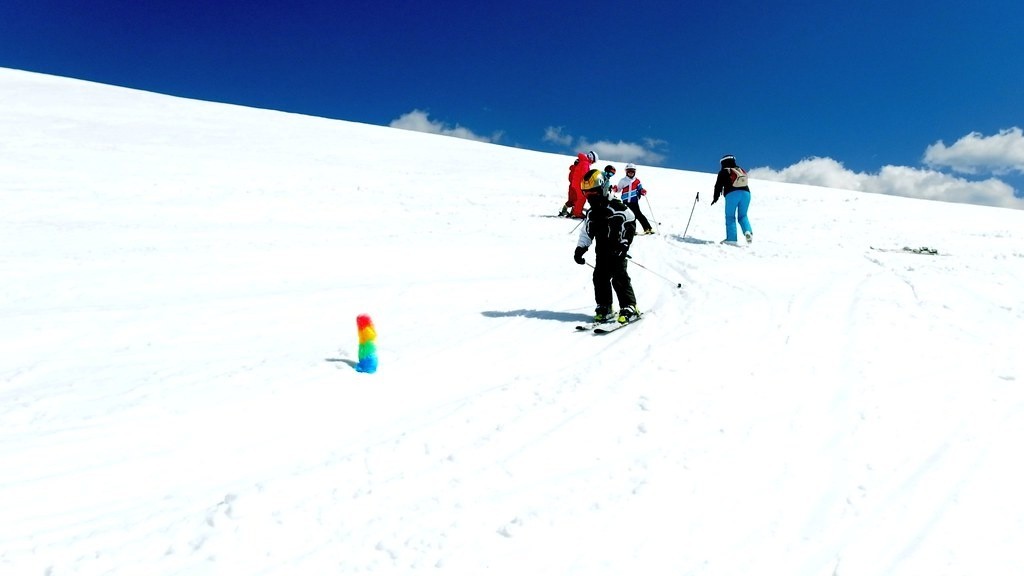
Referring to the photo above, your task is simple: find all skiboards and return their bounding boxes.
[633,229,654,236]
[575,311,644,334]
[534,213,587,219]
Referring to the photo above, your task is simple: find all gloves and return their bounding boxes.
[608,185,612,193]
[615,241,629,261]
[711,197,718,205]
[612,185,618,192]
[574,246,588,264]
[641,189,646,196]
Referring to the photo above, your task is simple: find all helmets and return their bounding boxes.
[581,169,610,203]
[720,155,736,162]
[604,165,615,177]
[625,163,636,169]
[587,150,598,163]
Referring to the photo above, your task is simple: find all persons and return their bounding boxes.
[599,165,615,197]
[560,160,586,216]
[610,162,655,234]
[572,168,642,323]
[571,150,595,216]
[711,154,756,246]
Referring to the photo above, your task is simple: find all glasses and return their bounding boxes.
[627,170,635,173]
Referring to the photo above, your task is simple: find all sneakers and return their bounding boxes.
[594,306,615,322]
[618,304,640,323]
[720,239,737,245]
[562,210,569,214]
[745,231,752,242]
[645,228,655,234]
